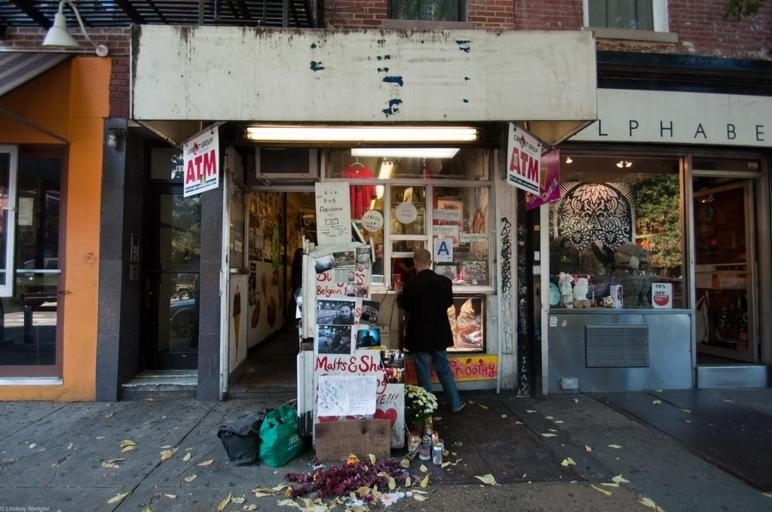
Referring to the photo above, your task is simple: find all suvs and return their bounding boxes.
[14,257,60,308]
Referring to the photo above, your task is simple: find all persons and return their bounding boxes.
[359,331,376,346]
[333,303,354,324]
[396,248,466,414]
[288,246,303,326]
[333,329,351,353]
[441,267,455,280]
[372,244,408,275]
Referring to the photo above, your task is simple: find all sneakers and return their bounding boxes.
[451,402,466,413]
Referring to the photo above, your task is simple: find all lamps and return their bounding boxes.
[40,0,109,57]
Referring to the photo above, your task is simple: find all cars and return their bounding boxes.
[168,272,199,340]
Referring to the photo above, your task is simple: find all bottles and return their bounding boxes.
[418,424,446,466]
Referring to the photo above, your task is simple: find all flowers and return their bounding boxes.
[404,384,439,426]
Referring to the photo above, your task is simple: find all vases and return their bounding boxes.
[407,424,423,437]
